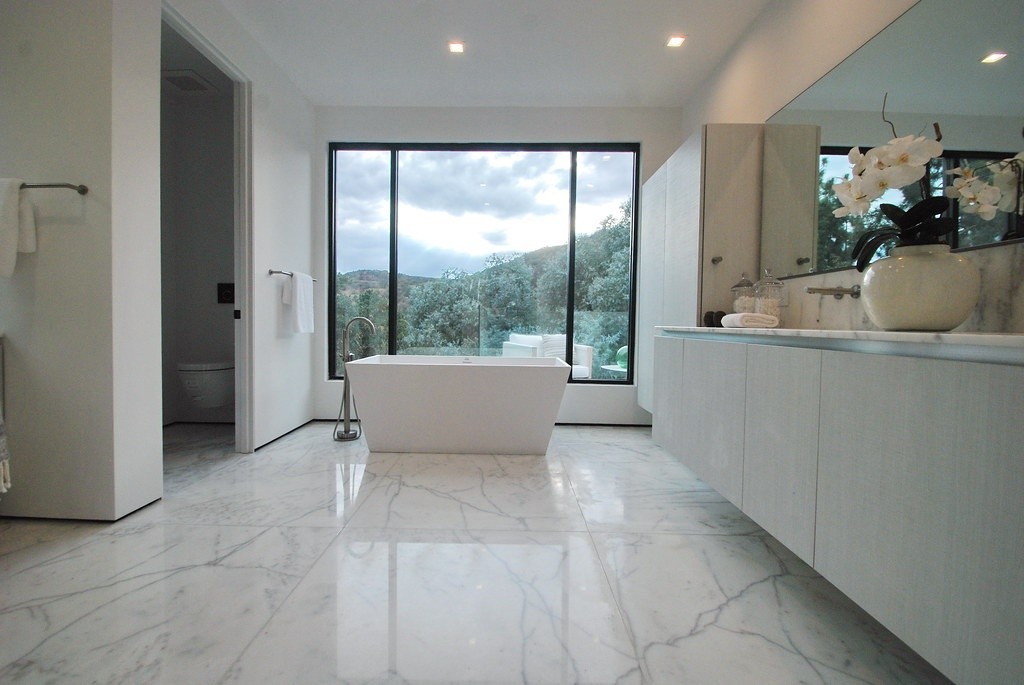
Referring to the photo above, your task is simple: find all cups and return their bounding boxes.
[753,269,788,329]
[731,273,754,313]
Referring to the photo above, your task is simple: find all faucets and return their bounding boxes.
[346,316,376,336]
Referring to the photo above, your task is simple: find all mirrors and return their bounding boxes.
[763,0,1024,280]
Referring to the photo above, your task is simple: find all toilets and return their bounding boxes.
[177,359,234,415]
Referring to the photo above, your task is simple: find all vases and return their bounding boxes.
[861,244,980,332]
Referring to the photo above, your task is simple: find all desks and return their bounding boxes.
[602,364,627,379]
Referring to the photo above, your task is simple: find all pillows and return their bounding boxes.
[543,334,579,365]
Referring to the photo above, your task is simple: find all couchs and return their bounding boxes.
[503,332,593,379]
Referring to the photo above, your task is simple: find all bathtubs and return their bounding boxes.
[345,354,571,456]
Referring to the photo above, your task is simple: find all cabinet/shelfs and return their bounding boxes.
[637,123,765,414]
[652,334,1024,684]
[760,122,822,280]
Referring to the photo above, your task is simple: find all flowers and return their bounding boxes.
[831,133,957,272]
[944,150,1024,240]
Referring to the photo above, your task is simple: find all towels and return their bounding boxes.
[1,176,37,279]
[720,313,780,327]
[282,271,314,336]
